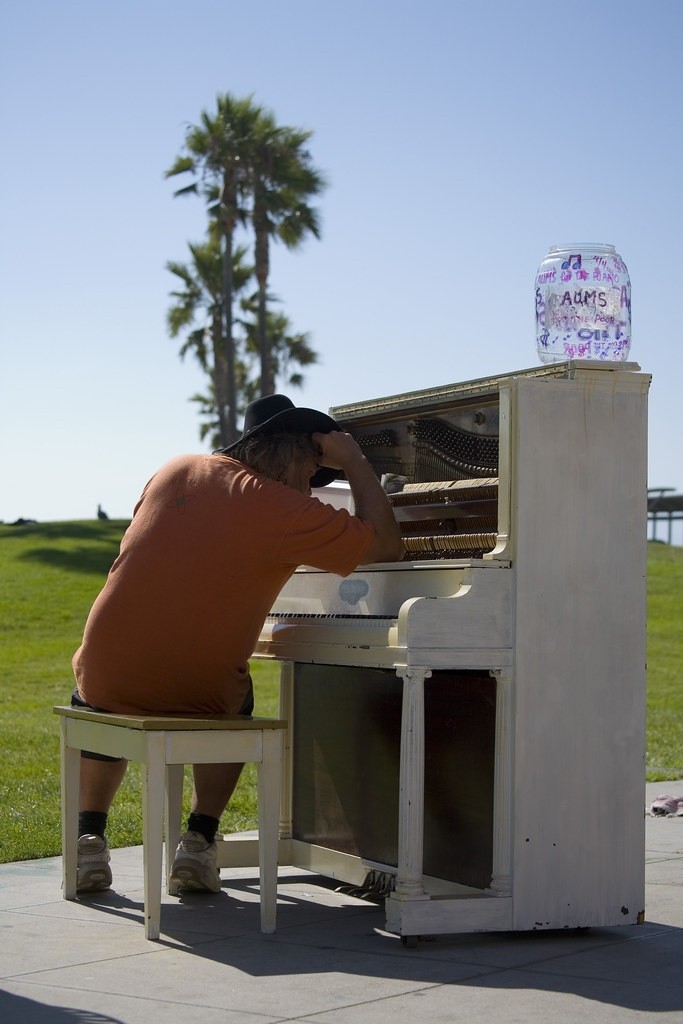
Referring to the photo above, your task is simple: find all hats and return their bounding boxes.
[212,394,345,488]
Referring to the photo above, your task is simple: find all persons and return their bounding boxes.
[70,392,407,896]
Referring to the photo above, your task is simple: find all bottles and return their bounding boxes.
[534,242,631,363]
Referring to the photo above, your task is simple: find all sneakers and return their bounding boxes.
[168,831,222,893]
[77,834,112,891]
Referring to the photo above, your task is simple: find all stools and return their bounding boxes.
[53,706,288,942]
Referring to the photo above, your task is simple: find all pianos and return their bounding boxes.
[246,357,654,937]
